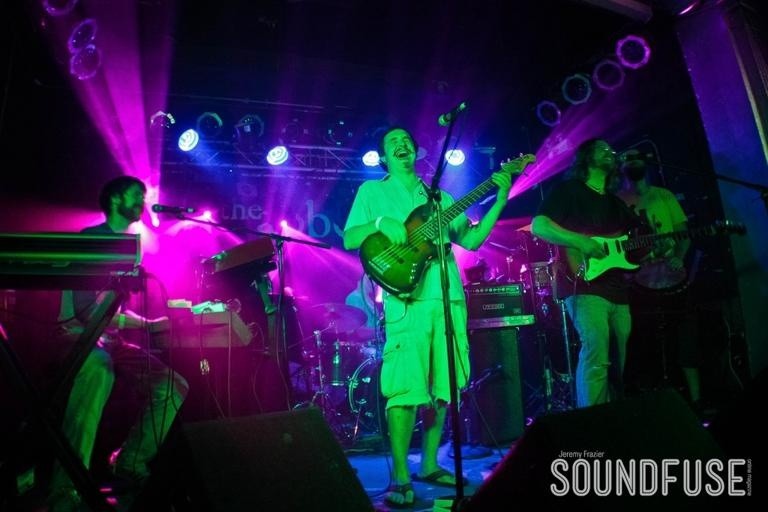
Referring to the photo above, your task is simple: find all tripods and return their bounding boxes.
[305,334,350,445]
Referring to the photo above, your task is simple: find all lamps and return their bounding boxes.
[146,101,470,184]
[537,30,658,139]
[33,0,105,83]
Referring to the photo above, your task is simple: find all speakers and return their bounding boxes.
[127,405,376,512]
[460,388,739,512]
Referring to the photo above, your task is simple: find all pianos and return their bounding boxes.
[1,235,139,292]
[141,313,254,349]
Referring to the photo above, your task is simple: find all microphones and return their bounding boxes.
[613,152,653,165]
[437,101,470,129]
[151,202,196,217]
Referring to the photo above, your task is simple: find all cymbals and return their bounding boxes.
[309,303,367,330]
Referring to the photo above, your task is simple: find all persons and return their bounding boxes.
[531,138,644,410]
[45,175,190,512]
[342,128,512,509]
[345,276,386,348]
[617,145,706,417]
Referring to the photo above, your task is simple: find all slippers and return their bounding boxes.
[409,465,468,488]
[382,478,416,509]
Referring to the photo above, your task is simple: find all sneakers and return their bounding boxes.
[111,463,152,487]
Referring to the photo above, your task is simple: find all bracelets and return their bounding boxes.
[374,216,382,230]
[117,313,126,330]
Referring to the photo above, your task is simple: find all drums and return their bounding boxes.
[520,262,554,298]
[325,346,371,387]
[347,357,425,430]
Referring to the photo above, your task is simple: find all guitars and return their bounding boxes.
[563,220,748,282]
[358,153,536,298]
